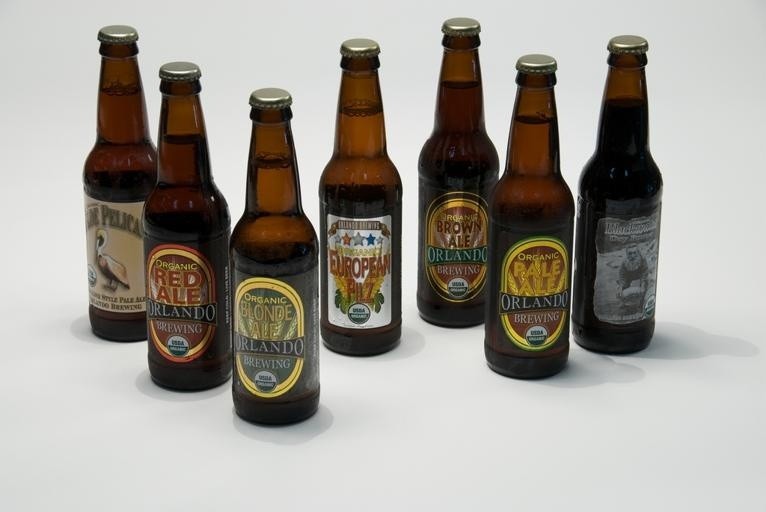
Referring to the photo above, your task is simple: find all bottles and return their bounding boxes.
[415,16,501,328]
[314,37,404,360]
[225,85,322,428]
[140,60,236,393]
[483,54,578,382]
[81,24,159,344]
[569,31,665,356]
